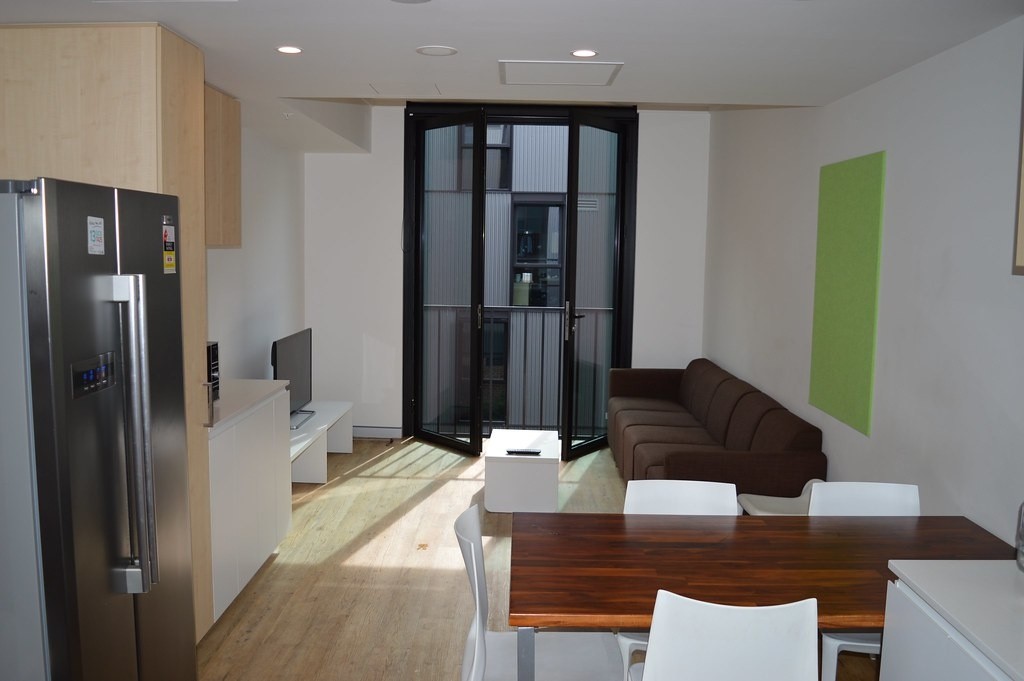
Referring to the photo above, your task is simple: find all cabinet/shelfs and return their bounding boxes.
[210,378,293,623]
[205,80,242,249]
[880,560,1024,681]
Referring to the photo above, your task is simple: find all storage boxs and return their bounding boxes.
[207,341,219,401]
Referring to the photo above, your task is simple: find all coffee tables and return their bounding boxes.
[483,428,561,512]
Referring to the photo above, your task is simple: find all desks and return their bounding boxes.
[509,513,1019,680]
[290,402,355,484]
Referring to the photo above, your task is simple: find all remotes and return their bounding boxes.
[507,449,541,455]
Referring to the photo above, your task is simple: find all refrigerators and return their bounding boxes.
[0,176,199,681]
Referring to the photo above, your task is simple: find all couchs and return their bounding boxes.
[609,358,828,498]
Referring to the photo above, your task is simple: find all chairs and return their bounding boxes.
[736,479,825,515]
[626,589,819,681]
[453,504,625,681]
[808,482,920,681]
[615,478,739,681]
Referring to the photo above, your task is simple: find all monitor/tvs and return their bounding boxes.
[272,328,316,430]
